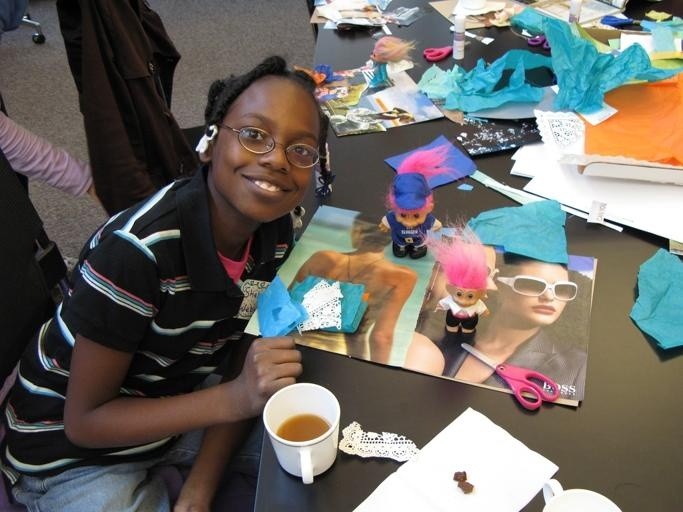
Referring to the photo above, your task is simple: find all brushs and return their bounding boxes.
[450,26,495,46]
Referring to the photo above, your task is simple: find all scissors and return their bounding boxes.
[528,35,550,50]
[601,15,643,26]
[460,341,560,411]
[423,41,470,61]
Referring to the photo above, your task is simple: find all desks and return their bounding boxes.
[254,0,683,512]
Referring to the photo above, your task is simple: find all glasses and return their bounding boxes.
[218,122,325,169]
[495,274,578,301]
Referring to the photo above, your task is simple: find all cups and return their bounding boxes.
[262,381,341,485]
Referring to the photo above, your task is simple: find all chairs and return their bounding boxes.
[0,148,182,509]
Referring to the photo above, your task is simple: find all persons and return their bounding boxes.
[361,36,416,96]
[419,216,494,335]
[287,213,419,364]
[0,54,334,512]
[378,140,465,258]
[407,257,587,399]
[0,95,99,201]
